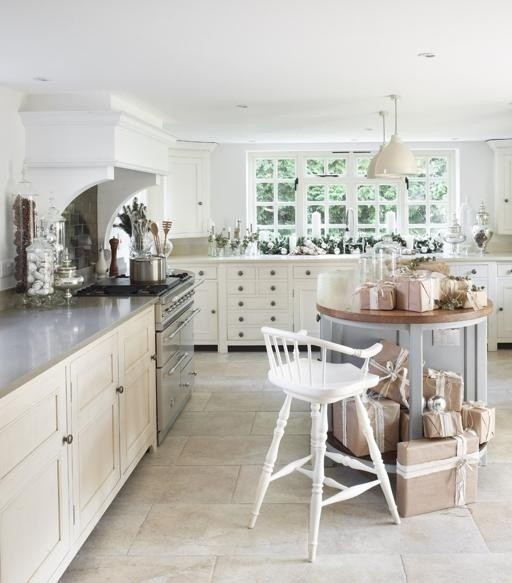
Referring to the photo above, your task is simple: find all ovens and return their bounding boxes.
[156,277,196,445]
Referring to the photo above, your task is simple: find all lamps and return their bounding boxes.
[366,94,426,180]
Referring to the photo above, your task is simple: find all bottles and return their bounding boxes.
[362,235,400,281]
[25,236,54,295]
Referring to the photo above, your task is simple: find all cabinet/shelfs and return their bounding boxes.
[293,263,321,345]
[222,262,291,347]
[119,304,154,493]
[448,262,490,343]
[0,344,70,582]
[72,323,120,561]
[184,262,220,346]
[487,138,512,236]
[495,262,512,343]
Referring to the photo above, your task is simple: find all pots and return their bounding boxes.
[129,251,166,284]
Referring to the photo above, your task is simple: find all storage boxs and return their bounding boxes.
[331,339,495,518]
[359,269,441,313]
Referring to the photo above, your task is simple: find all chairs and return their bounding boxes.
[248,326,402,561]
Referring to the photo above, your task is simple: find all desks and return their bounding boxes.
[316,296,494,437]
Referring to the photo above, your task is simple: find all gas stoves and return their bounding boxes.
[72,271,193,301]
